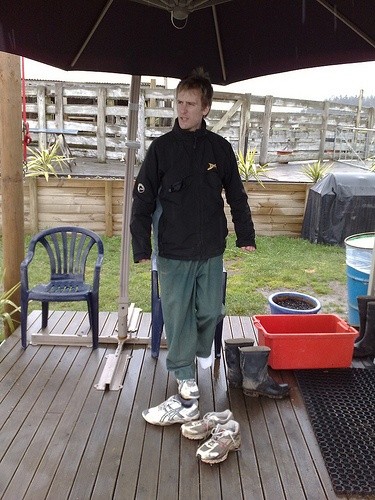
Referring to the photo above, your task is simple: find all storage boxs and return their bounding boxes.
[253,314,359,369]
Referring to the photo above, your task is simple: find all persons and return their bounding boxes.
[130,73,257,400]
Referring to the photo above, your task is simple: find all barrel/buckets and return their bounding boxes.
[344,232,375,327]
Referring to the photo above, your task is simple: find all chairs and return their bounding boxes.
[151,254,227,358]
[20,227,104,348]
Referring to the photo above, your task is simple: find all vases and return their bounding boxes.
[269,292,321,315]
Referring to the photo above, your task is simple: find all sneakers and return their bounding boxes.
[194,419,241,465]
[176,378,201,400]
[179,408,235,441]
[141,393,200,427]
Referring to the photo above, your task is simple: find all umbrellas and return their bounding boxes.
[0,0,375,340]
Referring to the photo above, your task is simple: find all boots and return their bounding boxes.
[224,337,255,391]
[351,301,375,358]
[355,296,375,342]
[237,344,291,399]
